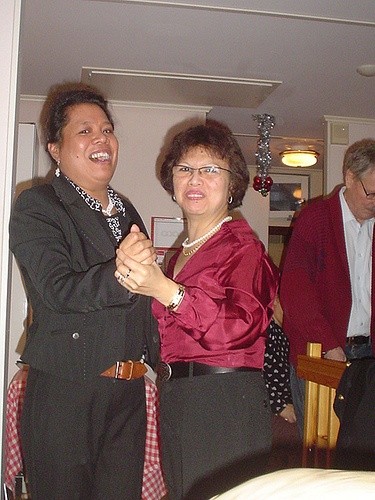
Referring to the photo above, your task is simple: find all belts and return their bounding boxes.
[102,360,149,379]
[347,335,372,347]
[157,360,261,384]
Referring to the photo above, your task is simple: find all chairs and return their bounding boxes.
[297,343,375,472]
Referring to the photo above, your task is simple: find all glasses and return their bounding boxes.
[359,176,375,203]
[169,165,232,179]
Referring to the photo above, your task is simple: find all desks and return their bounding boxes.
[4,366,166,500]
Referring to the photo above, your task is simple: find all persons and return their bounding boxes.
[113,124,279,500]
[280,138,375,440]
[263,291,303,467]
[9,84,159,500]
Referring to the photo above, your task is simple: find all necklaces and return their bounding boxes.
[181,216,232,256]
[102,196,114,216]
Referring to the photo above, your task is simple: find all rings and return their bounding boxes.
[126,269,131,277]
[117,274,124,281]
[285,418,288,421]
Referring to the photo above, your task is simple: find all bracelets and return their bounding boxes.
[167,285,183,309]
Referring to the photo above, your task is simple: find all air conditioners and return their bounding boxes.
[279,150,320,168]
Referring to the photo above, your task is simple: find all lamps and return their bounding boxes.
[357,65,375,77]
